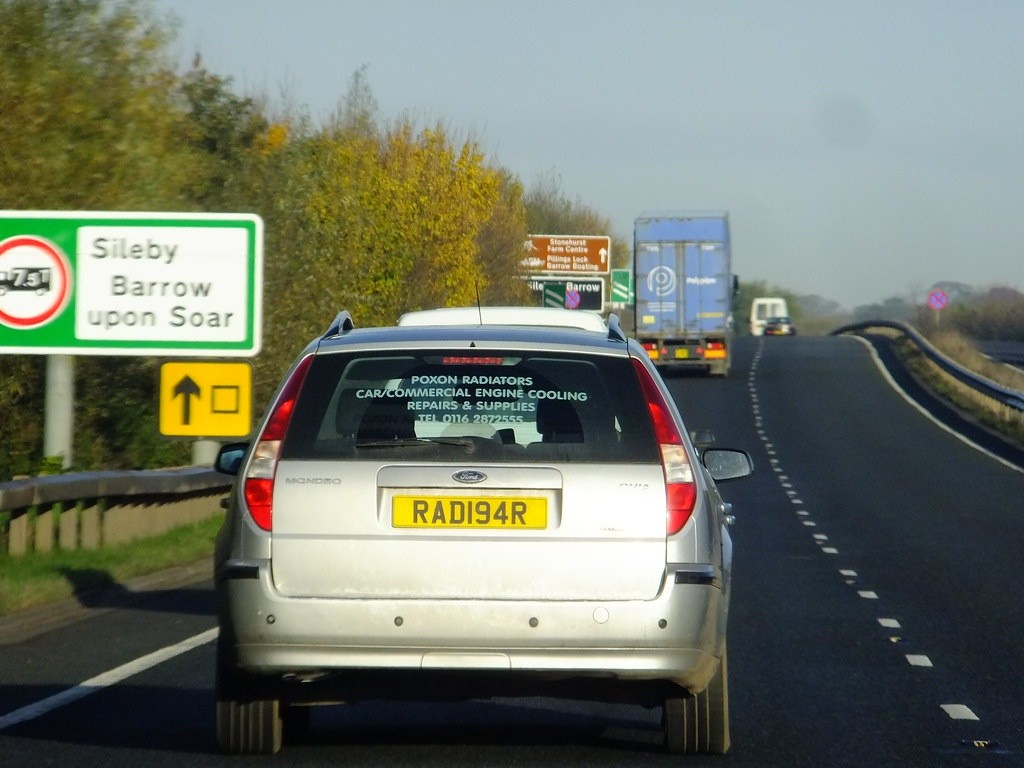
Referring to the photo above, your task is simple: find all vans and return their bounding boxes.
[747,296,785,336]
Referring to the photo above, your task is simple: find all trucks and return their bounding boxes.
[633,210,740,377]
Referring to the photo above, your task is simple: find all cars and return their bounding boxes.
[767,315,797,335]
[209,305,756,755]
